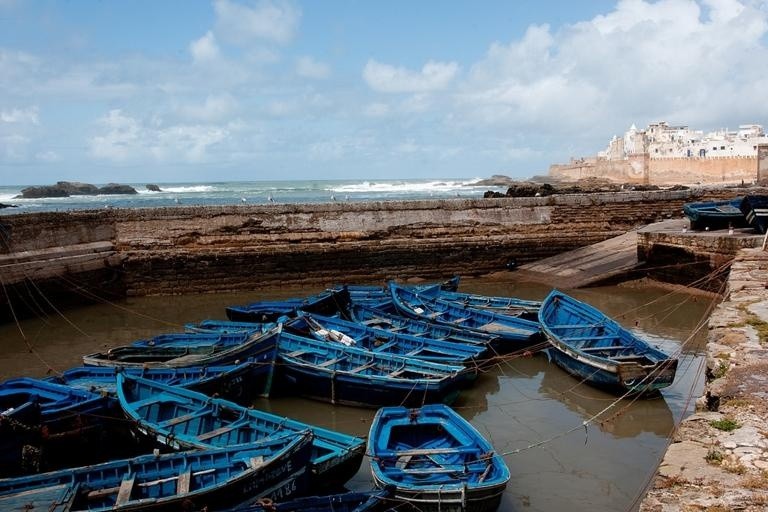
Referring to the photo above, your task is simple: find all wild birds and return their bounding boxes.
[103,204,113,209]
[268,195,275,203]
[241,197,247,204]
[345,195,349,201]
[174,197,182,206]
[330,194,336,201]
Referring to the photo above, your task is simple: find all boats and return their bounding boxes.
[683,193,768,234]
